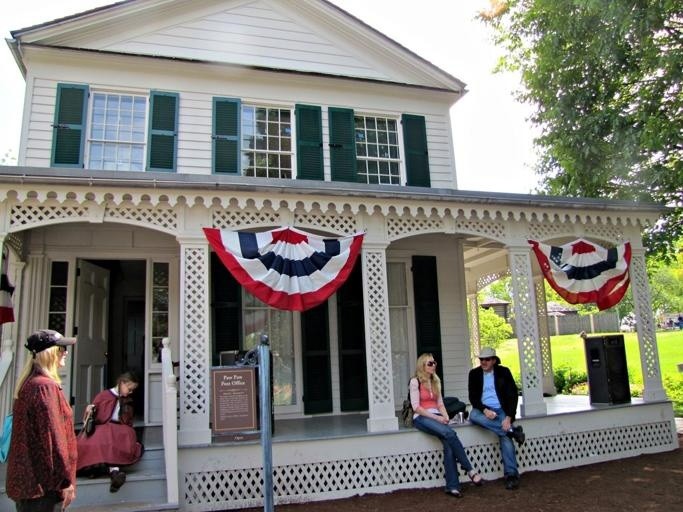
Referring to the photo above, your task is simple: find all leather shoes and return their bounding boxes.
[110,471,125,493]
[449,491,464,498]
[468,473,486,486]
[506,477,519,489]
[513,426,525,445]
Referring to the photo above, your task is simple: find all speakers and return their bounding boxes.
[585,334,632,405]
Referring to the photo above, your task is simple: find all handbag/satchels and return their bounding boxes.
[403,399,413,427]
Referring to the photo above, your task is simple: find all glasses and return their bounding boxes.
[479,358,491,361]
[427,361,437,366]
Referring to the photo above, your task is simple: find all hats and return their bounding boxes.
[24,330,78,353]
[475,347,500,364]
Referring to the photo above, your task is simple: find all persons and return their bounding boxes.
[403,350,489,497]
[2,327,81,511]
[77,368,147,495]
[464,350,528,491]
